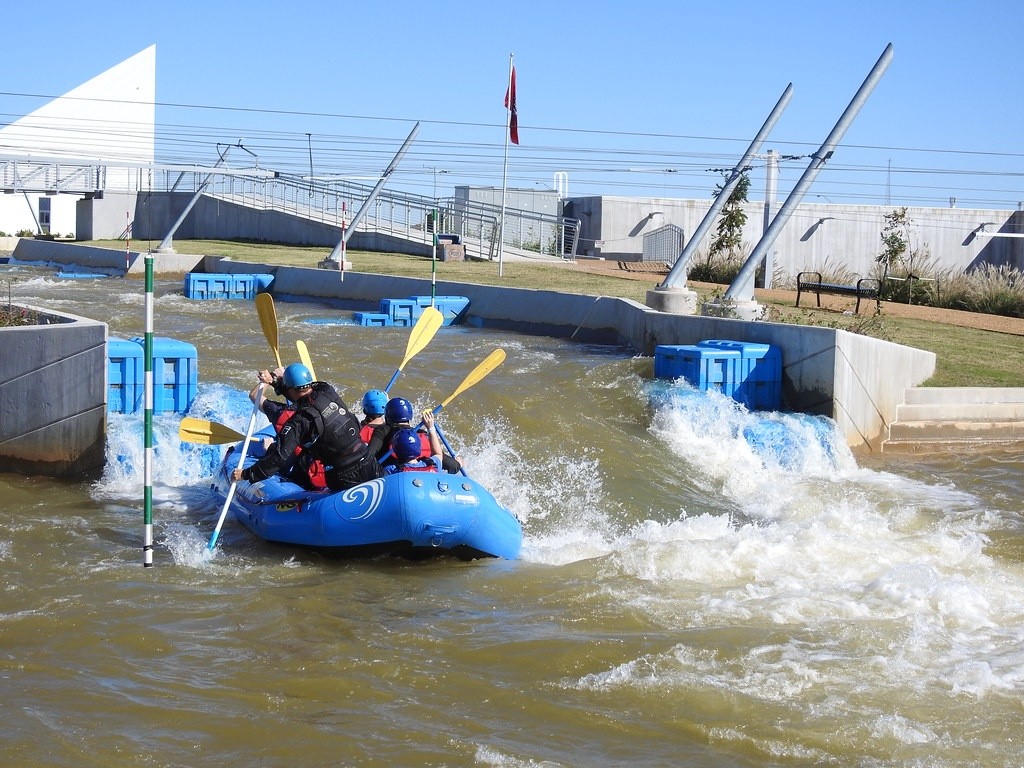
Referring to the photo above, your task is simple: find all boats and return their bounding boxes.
[181,382,522,562]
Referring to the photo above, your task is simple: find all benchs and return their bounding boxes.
[795,271,882,316]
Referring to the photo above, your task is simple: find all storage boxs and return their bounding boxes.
[185,272,254,300]
[436,234,467,262]
[352,298,415,327]
[108,335,198,416]
[654,339,783,413]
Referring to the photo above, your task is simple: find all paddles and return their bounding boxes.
[383,304,445,394]
[379,346,508,463]
[207,372,266,553]
[421,405,467,479]
[177,416,279,446]
[295,338,320,385]
[255,290,285,372]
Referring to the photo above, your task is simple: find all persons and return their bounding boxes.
[230,363,465,493]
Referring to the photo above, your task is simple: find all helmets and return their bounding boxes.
[392,429,421,459]
[362,390,388,414]
[283,363,313,388]
[385,398,413,424]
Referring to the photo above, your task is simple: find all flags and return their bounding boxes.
[505,65,519,144]
